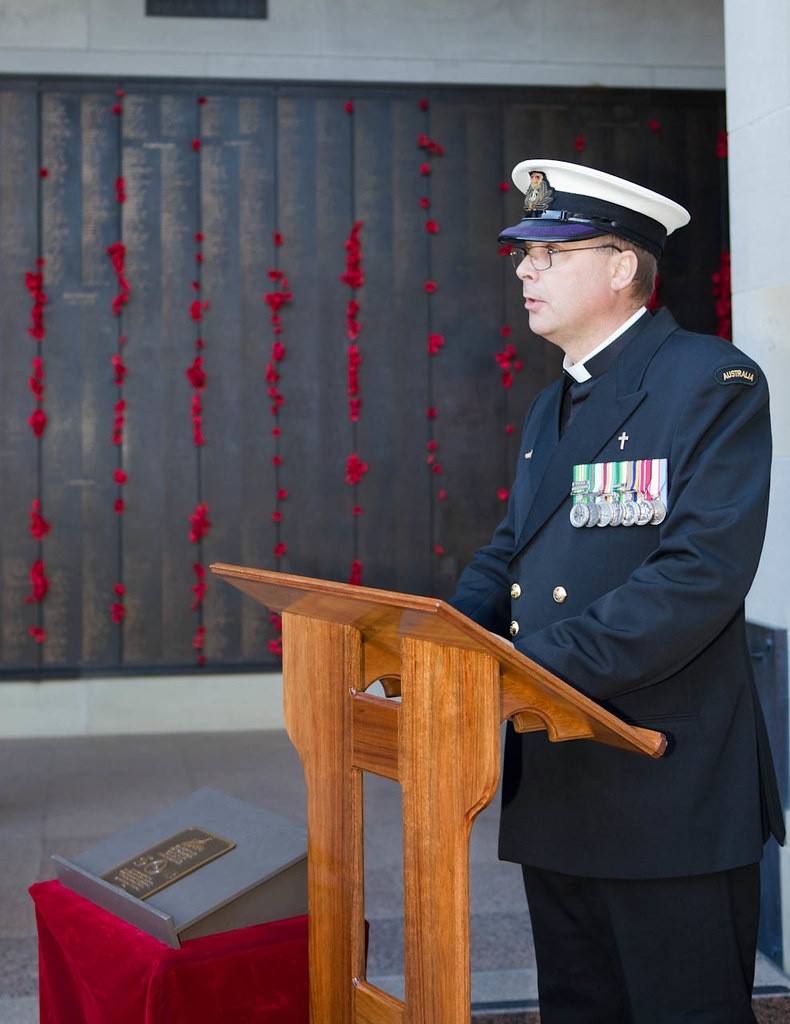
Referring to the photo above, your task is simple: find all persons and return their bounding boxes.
[449,157,785,1024]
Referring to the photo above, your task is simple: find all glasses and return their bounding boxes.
[509,245,623,271]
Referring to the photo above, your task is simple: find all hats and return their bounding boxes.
[496,158,691,262]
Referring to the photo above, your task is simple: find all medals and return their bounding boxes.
[570,492,666,527]
